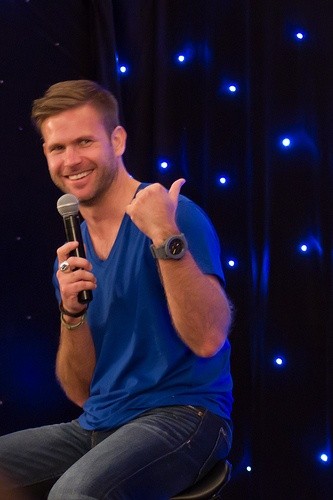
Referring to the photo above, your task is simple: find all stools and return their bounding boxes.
[169,460,232,500]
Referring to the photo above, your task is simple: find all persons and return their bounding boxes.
[0,81,234,500]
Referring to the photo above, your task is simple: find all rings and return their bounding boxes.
[60,261,71,273]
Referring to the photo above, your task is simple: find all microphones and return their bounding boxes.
[57,194,93,304]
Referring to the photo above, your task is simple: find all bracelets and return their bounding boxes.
[60,300,89,318]
[61,312,87,329]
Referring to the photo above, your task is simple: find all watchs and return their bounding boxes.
[150,233,188,259]
